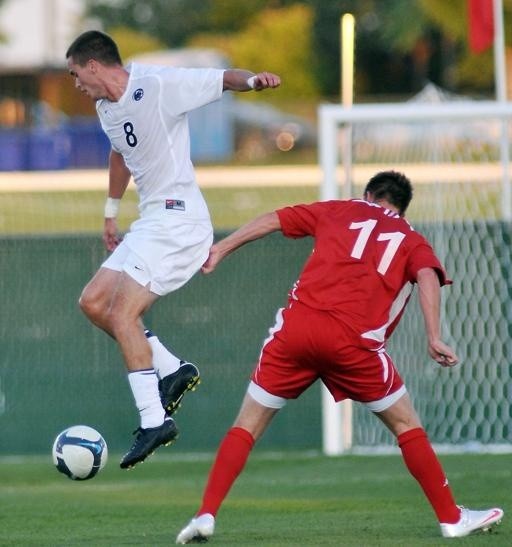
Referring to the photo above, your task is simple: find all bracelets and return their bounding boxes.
[103,195,122,219]
[245,73,260,89]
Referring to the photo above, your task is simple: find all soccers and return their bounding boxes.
[52,425,108,481]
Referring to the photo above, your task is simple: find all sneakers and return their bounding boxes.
[156,360,200,419]
[438,504,504,540]
[118,416,180,471]
[176,513,216,545]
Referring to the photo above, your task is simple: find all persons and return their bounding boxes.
[171,169,505,547]
[61,25,284,471]
